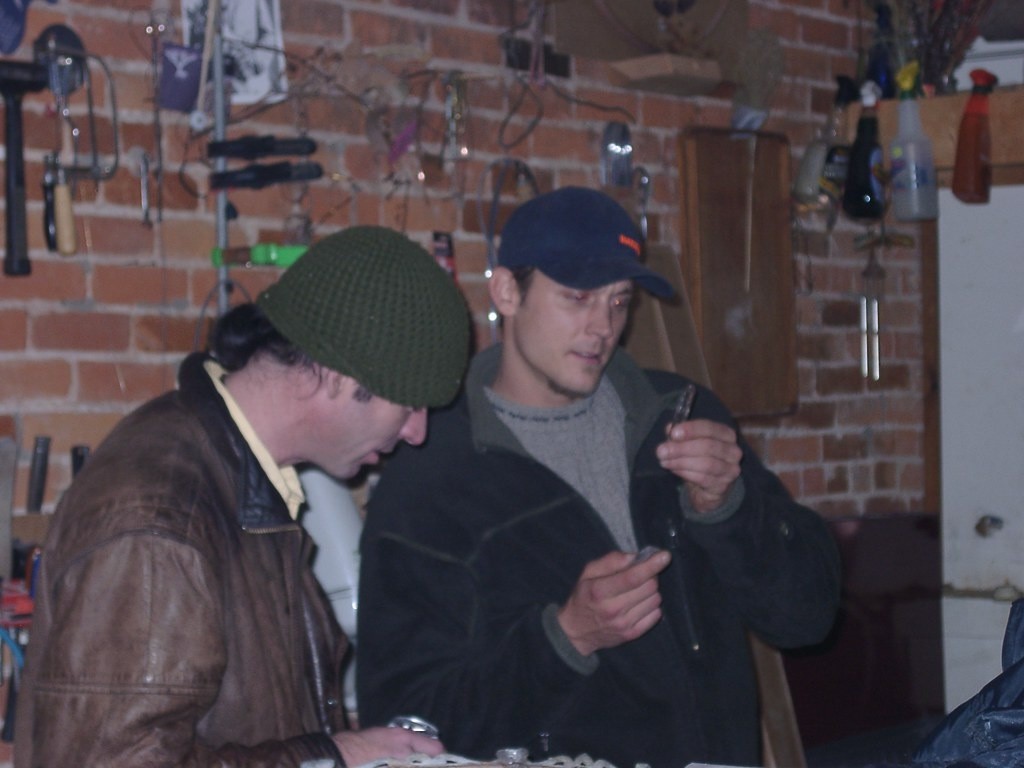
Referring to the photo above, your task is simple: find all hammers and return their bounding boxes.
[1,59,50,278]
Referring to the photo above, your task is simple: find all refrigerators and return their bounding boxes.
[938,191,1024,710]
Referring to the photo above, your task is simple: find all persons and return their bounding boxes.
[13,224,472,768]
[351,185,843,768]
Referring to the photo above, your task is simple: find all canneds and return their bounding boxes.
[388,715,439,741]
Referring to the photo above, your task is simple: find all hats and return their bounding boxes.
[255,225,471,406]
[500,185,685,308]
